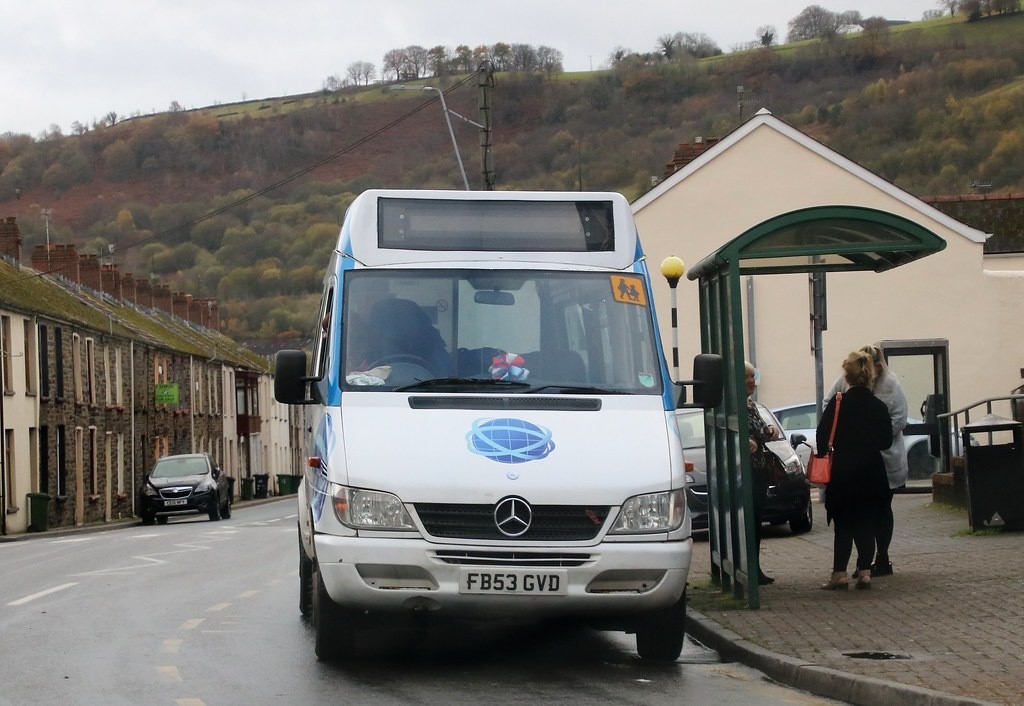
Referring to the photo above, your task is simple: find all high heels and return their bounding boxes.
[820,571,849,591]
[854,576,872,590]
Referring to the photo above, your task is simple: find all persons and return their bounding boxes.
[823,345,908,577]
[816,351,893,590]
[745,362,776,584]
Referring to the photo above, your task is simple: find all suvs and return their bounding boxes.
[139,453,233,525]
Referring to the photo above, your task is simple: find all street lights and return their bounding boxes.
[390,84,470,189]
[660,257,686,379]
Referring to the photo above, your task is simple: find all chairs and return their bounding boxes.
[522,350,586,382]
[359,297,456,382]
[678,422,694,447]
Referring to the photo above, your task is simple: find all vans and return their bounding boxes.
[274,188,724,662]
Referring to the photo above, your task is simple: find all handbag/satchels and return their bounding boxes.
[808,454,832,484]
[764,452,788,486]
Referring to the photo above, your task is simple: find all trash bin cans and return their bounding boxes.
[290,475,303,493]
[253,474,269,499]
[226,476,235,503]
[240,478,254,500]
[25,492,51,533]
[961,413,1024,533]
[276,474,291,495]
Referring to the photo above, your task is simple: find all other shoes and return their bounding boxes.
[758,568,775,585]
[871,563,893,577]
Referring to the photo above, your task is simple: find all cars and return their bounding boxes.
[771,401,982,478]
[670,403,812,535]
